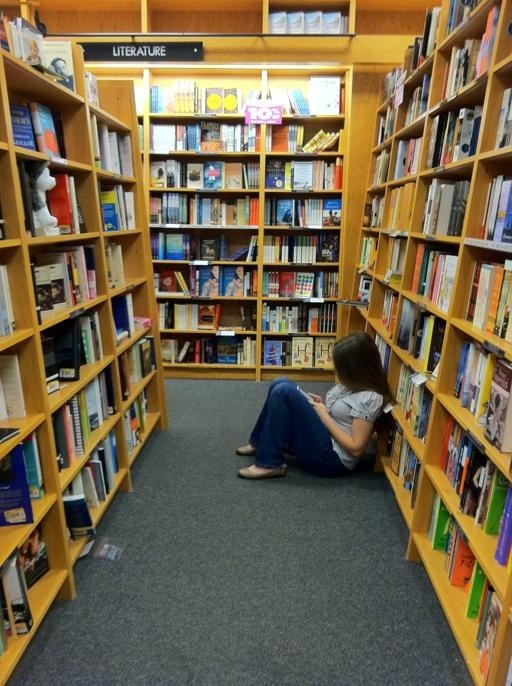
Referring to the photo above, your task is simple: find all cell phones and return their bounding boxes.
[297,385,315,403]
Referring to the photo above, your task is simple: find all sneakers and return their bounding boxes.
[238,464,287,479]
[236,445,256,456]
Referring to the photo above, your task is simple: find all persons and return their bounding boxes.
[236,330,396,480]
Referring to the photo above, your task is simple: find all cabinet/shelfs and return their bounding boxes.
[0,1,359,52]
[351,1,511,685]
[137,70,343,380]
[1,17,167,685]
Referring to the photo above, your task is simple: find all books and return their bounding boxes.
[155,73,347,372]
[0,9,157,660]
[357,0,512,686]
